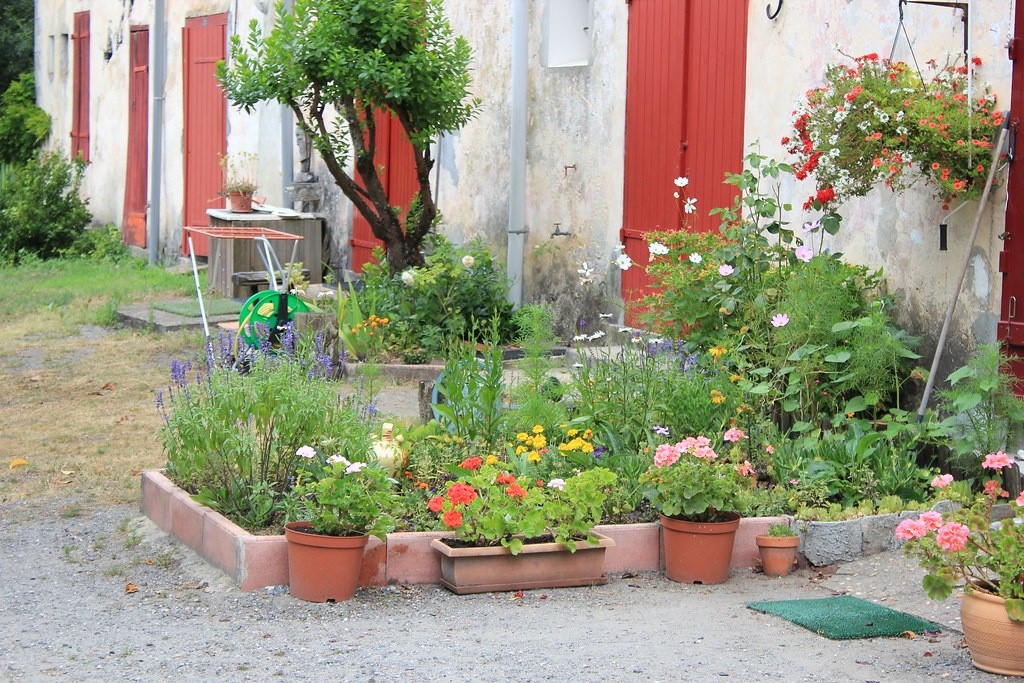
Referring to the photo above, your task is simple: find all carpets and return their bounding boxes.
[154,299,243,317]
[747,594,940,640]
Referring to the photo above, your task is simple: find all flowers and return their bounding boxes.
[274,444,401,543]
[781,53,1007,215]
[426,424,618,556]
[897,450,1024,622]
[640,427,757,524]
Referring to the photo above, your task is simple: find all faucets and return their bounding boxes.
[549,222,571,239]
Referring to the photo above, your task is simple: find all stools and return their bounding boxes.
[231,270,310,306]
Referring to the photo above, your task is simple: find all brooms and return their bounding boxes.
[866,112,1010,477]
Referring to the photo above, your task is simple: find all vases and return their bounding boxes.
[282,520,370,604]
[657,509,740,585]
[961,579,1024,677]
[430,530,616,596]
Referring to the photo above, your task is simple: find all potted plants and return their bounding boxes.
[754,521,800,578]
[206,151,263,214]
